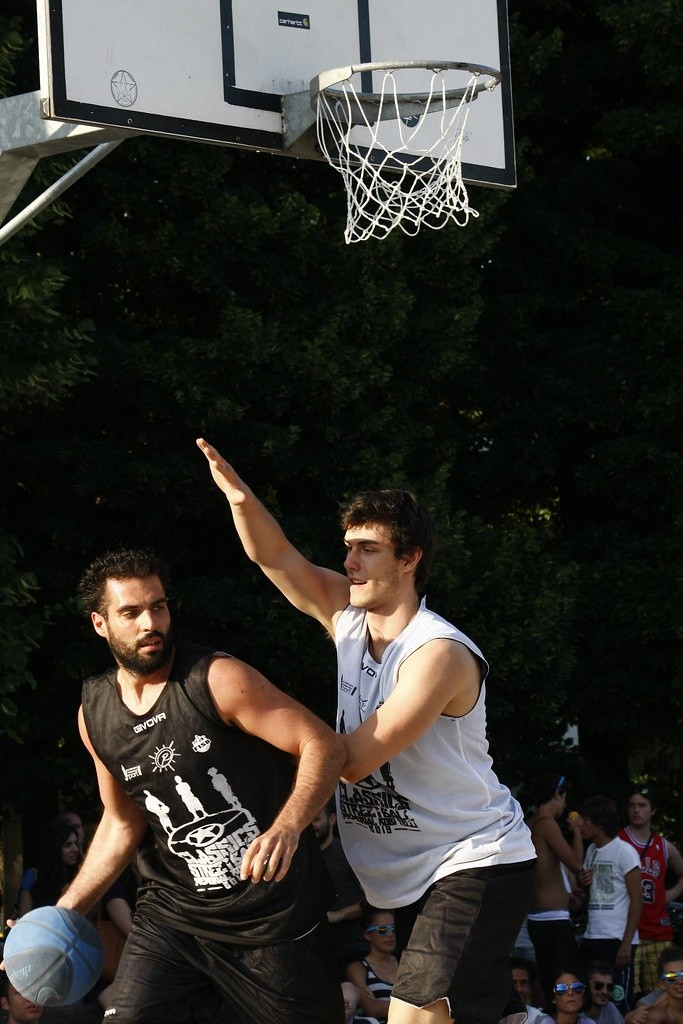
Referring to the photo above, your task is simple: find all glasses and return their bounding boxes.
[557,776,567,787]
[661,972,683,983]
[553,982,585,997]
[593,982,615,992]
[634,788,652,796]
[366,924,396,935]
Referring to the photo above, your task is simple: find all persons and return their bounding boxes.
[0,548,346,1024]
[194,437,539,1024]
[0,769,683,1024]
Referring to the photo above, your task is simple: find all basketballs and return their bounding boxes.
[3,906,103,1006]
